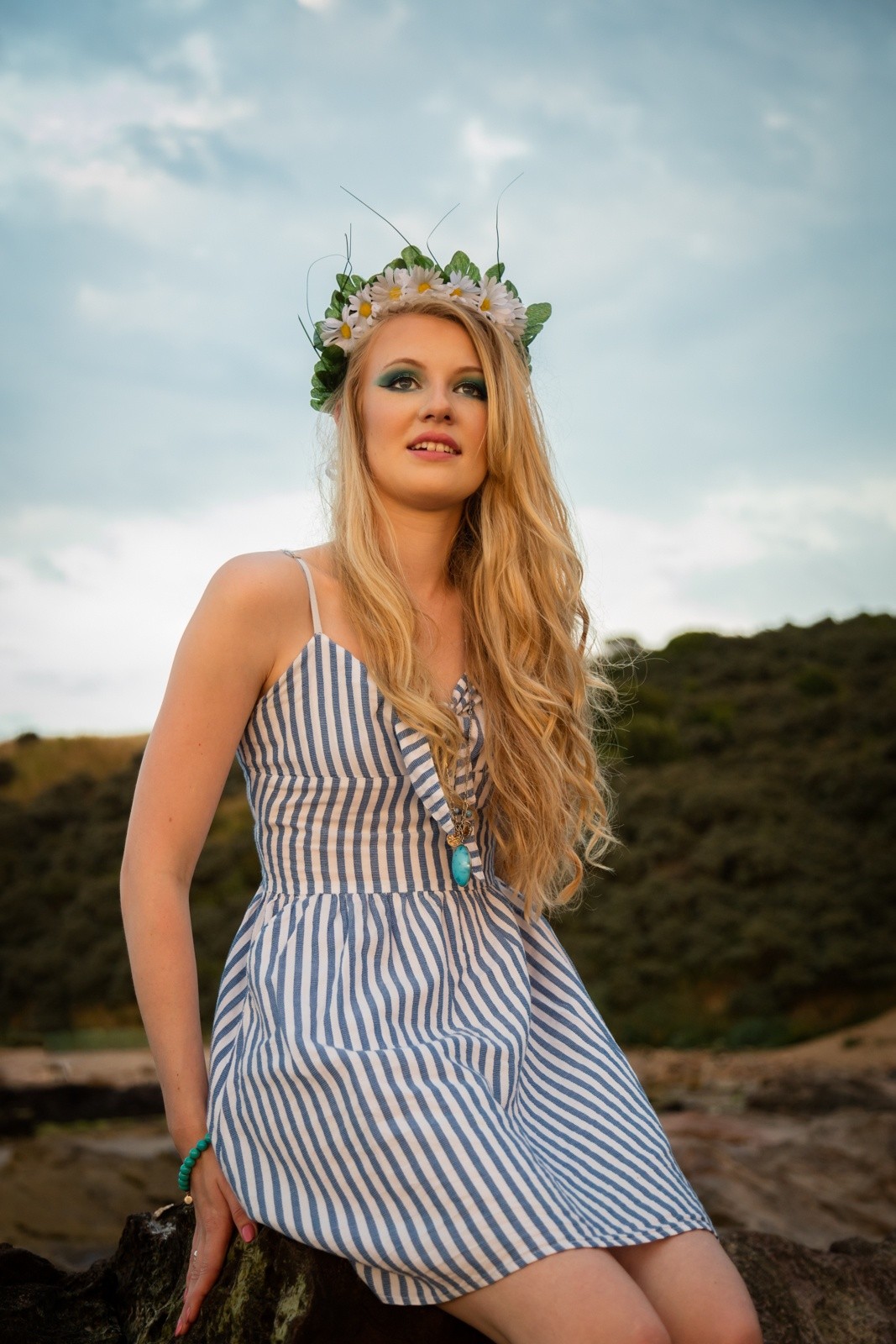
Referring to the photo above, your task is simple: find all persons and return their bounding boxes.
[119,244,764,1344]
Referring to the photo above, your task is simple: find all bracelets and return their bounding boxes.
[177,1133,213,1205]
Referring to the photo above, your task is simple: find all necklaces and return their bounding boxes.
[421,621,475,886]
